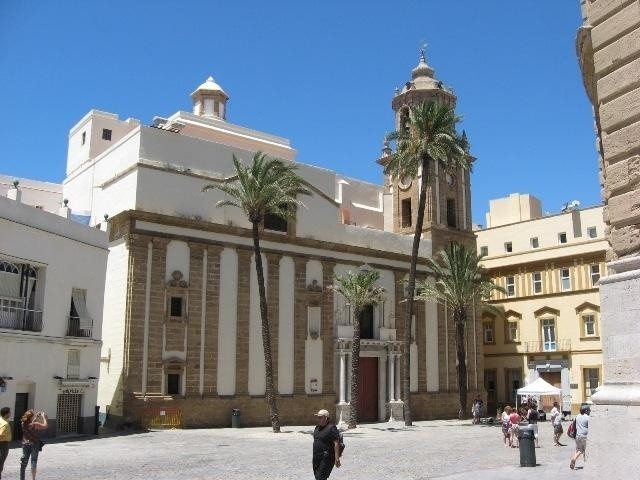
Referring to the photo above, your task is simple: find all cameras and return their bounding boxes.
[38,413,42,416]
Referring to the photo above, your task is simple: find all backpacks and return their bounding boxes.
[529,409,540,420]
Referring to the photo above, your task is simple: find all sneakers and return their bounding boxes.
[504,443,519,448]
[570,459,575,469]
[554,442,562,447]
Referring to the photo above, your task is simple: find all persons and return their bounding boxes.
[0,406,13,480]
[474,399,485,424]
[18,409,48,480]
[496,396,591,470]
[312,409,343,480]
[471,398,478,425]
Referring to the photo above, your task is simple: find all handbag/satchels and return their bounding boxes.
[567,421,576,438]
[39,441,44,451]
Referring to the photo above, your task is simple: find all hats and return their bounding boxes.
[314,409,330,418]
[581,404,591,412]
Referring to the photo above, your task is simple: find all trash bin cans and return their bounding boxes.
[232,408,241,428]
[518,430,536,467]
